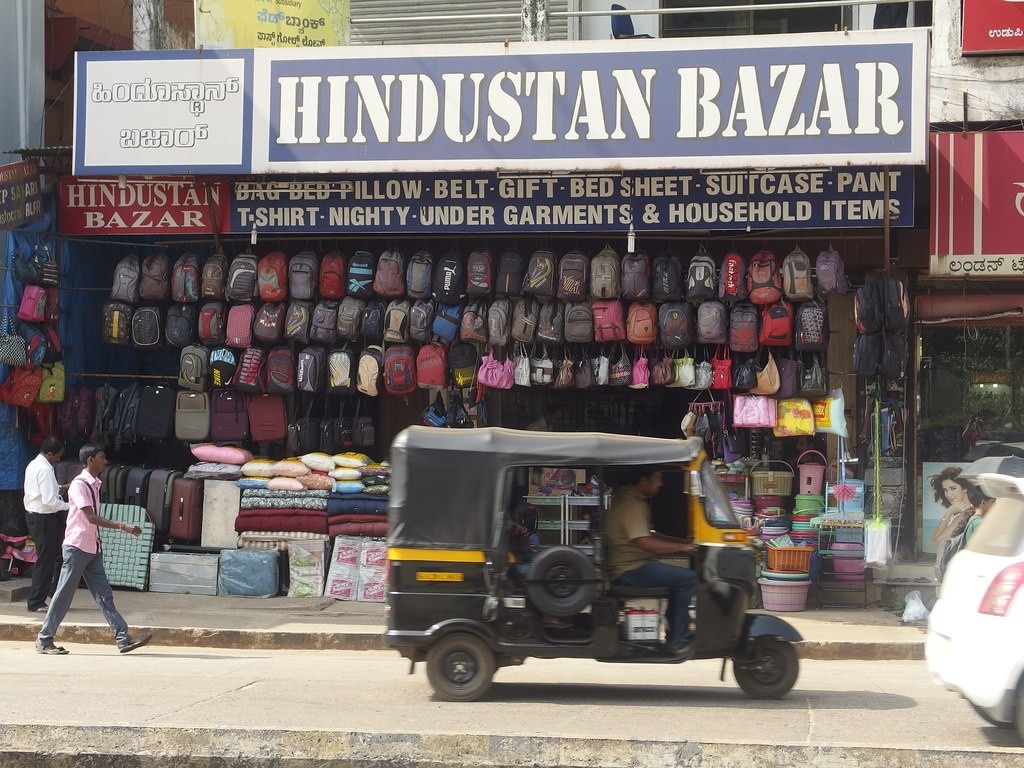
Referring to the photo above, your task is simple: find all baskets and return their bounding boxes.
[765,545,812,572]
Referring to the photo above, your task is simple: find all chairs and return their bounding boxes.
[590,510,671,600]
[611,4,654,39]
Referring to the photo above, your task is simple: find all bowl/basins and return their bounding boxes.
[757,567,812,612]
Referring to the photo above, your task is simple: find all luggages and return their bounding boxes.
[220,548,281,598]
[52,463,206,549]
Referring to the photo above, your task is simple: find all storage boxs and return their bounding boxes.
[150,551,220,596]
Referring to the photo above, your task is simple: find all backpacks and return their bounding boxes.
[936,515,984,586]
[0,235,913,469]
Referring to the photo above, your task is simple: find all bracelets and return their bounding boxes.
[941,517,951,523]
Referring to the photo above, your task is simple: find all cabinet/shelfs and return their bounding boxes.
[524,493,612,560]
[817,542,871,612]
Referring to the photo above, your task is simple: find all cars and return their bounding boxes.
[924,454,1024,748]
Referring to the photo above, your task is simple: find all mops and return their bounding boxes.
[864,401,892,565]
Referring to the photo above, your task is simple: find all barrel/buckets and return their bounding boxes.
[796,450,827,495]
[623,598,661,644]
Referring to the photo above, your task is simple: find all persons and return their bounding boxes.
[926,466,974,547]
[601,466,693,653]
[510,521,593,644]
[34,443,152,655]
[23,438,70,613]
[964,485,996,542]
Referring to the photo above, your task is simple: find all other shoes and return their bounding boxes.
[672,643,693,658]
[33,606,48,613]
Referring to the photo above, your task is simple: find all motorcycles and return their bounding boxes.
[382,424,805,702]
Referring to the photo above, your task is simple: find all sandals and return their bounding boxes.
[118,633,151,651]
[35,641,70,655]
